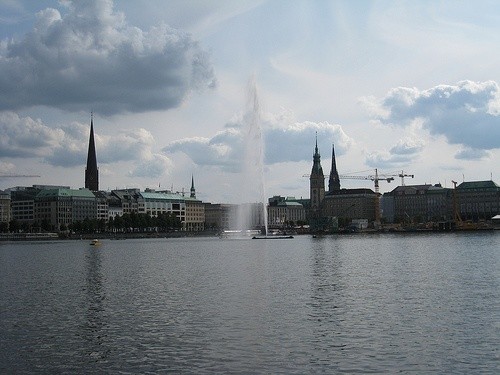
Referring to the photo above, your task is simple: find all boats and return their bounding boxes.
[472,222,494,230]
[252,235,294,239]
[90,239,101,246]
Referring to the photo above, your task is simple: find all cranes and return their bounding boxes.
[302,169,458,226]
